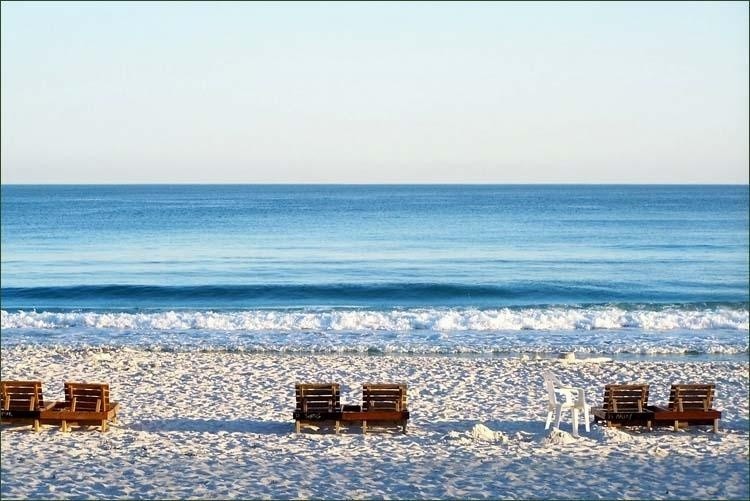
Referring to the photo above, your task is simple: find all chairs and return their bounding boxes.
[542,371,592,437]
[652,384,722,434]
[589,384,655,433]
[342,384,411,436]
[0,380,55,433]
[293,382,347,436]
[40,381,120,433]
